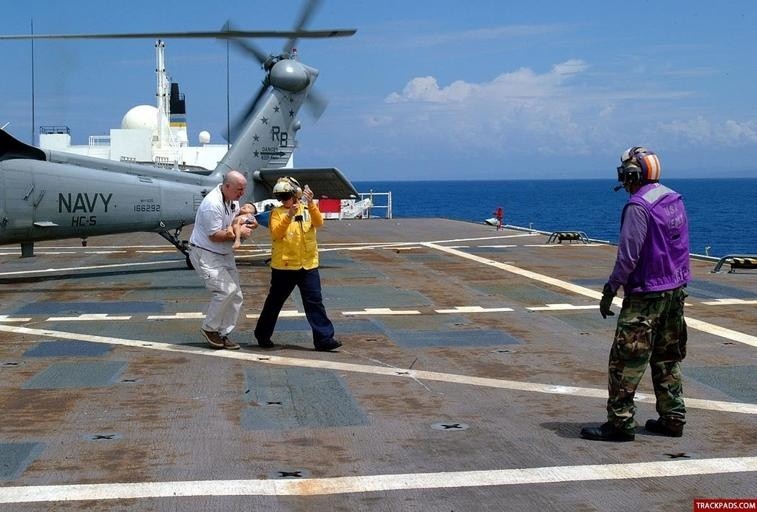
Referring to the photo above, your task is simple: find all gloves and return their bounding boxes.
[599,291,615,320]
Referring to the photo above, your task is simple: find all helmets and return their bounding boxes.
[613,145,661,192]
[272,175,302,202]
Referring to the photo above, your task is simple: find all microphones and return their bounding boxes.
[613,184,625,192]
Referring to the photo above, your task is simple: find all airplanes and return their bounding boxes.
[0,1,362,258]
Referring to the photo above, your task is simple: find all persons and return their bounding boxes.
[581,146,691,443]
[226,199,258,249]
[187,170,253,350]
[252,175,343,353]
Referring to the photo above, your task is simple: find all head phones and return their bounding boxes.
[277,177,302,198]
[623,146,640,187]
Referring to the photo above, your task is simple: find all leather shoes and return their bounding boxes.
[314,338,343,351]
[645,416,684,438]
[254,329,275,348]
[580,421,635,442]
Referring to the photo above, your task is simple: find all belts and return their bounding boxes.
[190,244,229,256]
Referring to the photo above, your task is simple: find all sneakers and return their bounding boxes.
[199,328,225,348]
[219,335,240,350]
